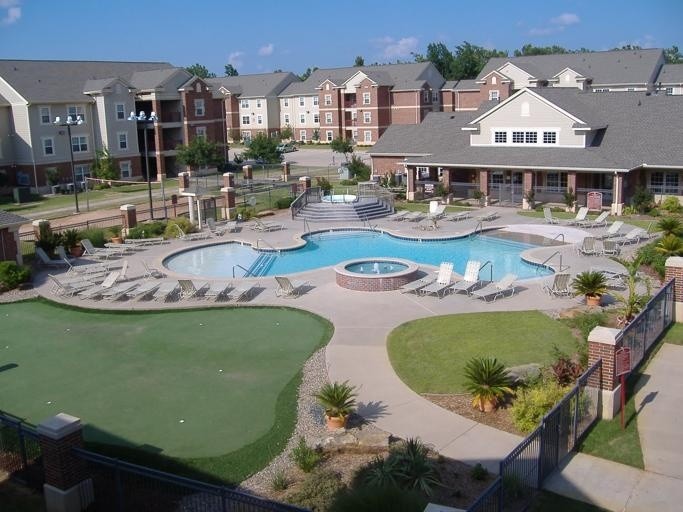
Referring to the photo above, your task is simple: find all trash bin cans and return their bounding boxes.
[67,184,74,193]
[13,187,31,203]
[80,182,86,189]
[52,186,59,195]
[415,184,424,200]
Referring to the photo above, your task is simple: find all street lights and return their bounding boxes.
[127,111,160,220]
[53,116,84,215]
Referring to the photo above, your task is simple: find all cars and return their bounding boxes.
[276,143,296,153]
[255,157,281,165]
[217,163,242,172]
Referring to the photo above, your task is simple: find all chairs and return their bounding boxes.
[274,275,311,299]
[400,259,519,303]
[174,217,283,240]
[37,236,164,267]
[444,211,470,221]
[566,207,644,256]
[47,258,258,302]
[543,207,558,224]
[387,209,422,223]
[425,205,446,219]
[543,273,570,298]
[477,210,497,221]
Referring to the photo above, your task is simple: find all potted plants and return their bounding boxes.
[315,380,354,429]
[610,254,646,328]
[571,270,606,306]
[64,228,81,256]
[108,225,123,244]
[463,355,516,411]
